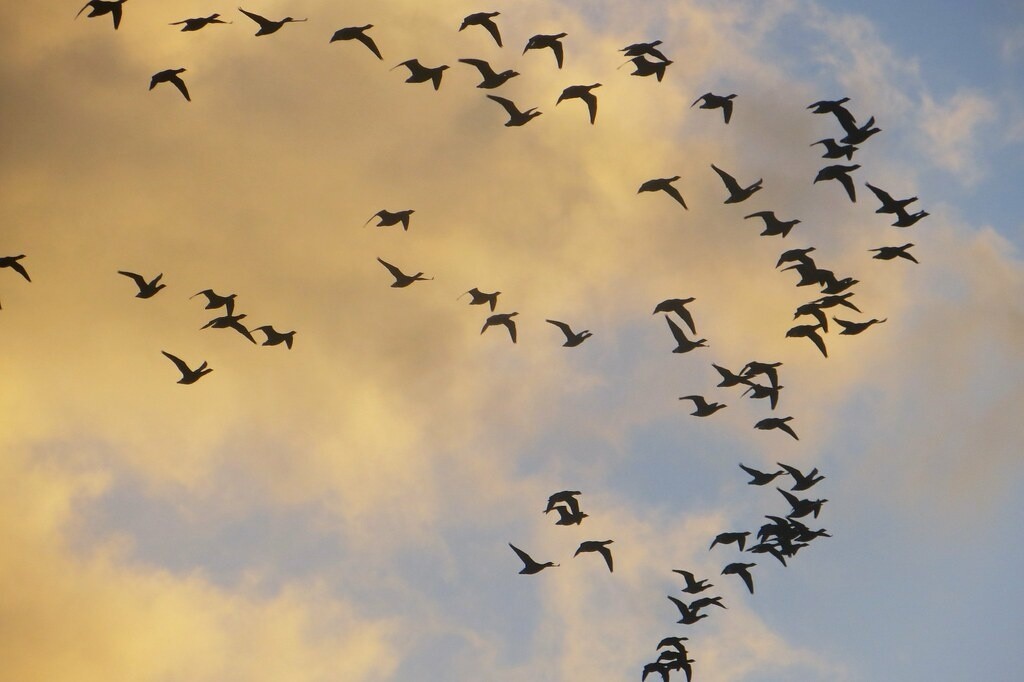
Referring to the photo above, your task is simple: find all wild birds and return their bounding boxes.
[458,12,504,48]
[377,258,434,289]
[250,325,297,350]
[76,0,127,28]
[505,33,925,682]
[237,7,308,37]
[329,23,384,60]
[458,288,501,312]
[200,313,257,345]
[457,59,520,89]
[190,289,237,317]
[0,254,31,308]
[481,312,518,344]
[391,58,449,91]
[546,319,593,348]
[116,269,166,299]
[149,68,191,102]
[169,13,227,32]
[363,209,414,231]
[160,350,213,384]
